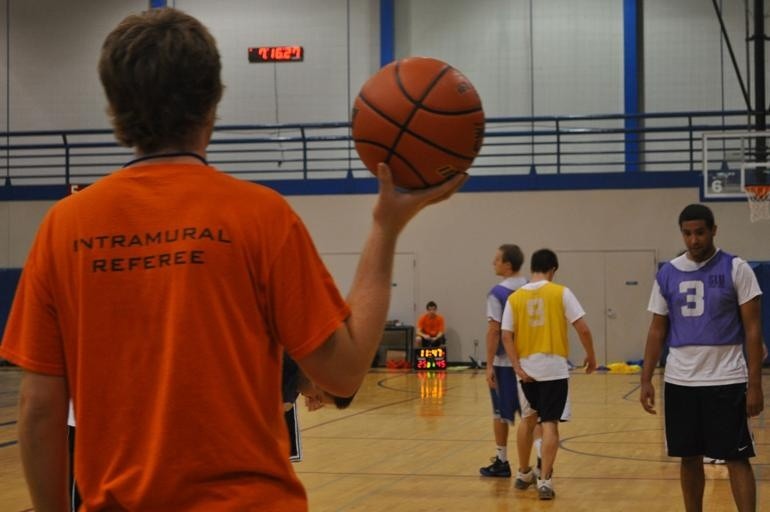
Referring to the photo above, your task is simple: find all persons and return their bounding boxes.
[64,395,86,510]
[416,300,446,348]
[278,346,359,418]
[501,247,599,501]
[637,204,763,512]
[0,5,472,510]
[477,241,554,481]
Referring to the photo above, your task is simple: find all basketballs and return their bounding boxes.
[352,58,484,192]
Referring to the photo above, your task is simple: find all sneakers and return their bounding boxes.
[513,467,536,488]
[537,459,555,500]
[479,457,510,477]
